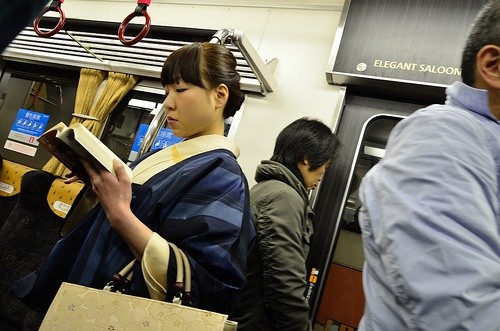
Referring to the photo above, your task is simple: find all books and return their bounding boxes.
[34,122,133,189]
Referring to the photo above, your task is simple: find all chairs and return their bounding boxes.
[0,156,39,229]
[0,170,85,331]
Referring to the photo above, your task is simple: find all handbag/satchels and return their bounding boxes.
[38,242,238,331]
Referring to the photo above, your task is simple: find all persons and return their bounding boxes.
[9,42,269,323]
[353,0,500,330]
[250,118,342,331]
